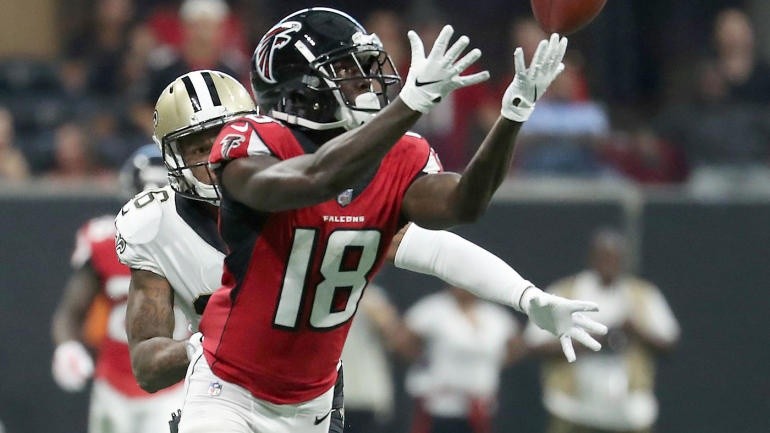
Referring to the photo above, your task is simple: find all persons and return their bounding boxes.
[0,0,770,199]
[52,143,192,433]
[399,285,522,433]
[326,283,408,433]
[114,70,608,433]
[168,7,569,433]
[523,223,682,433]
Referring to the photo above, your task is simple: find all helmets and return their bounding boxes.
[120,145,173,199]
[151,68,255,208]
[249,7,404,134]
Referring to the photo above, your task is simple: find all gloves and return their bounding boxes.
[500,31,567,124]
[519,285,609,364]
[52,340,96,395]
[399,25,490,114]
